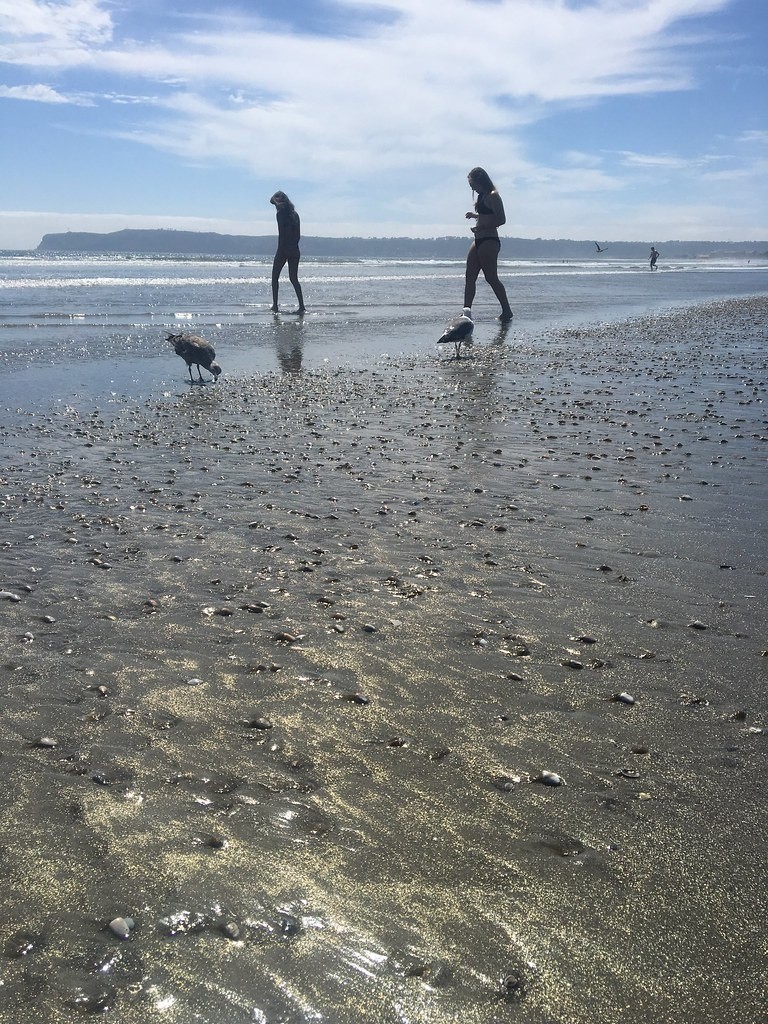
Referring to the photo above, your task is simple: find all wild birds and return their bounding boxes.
[161,330,222,383]
[595,242,608,253]
[436,307,474,361]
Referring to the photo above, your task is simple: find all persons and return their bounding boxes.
[648,247,659,271]
[269,191,306,314]
[463,167,513,322]
